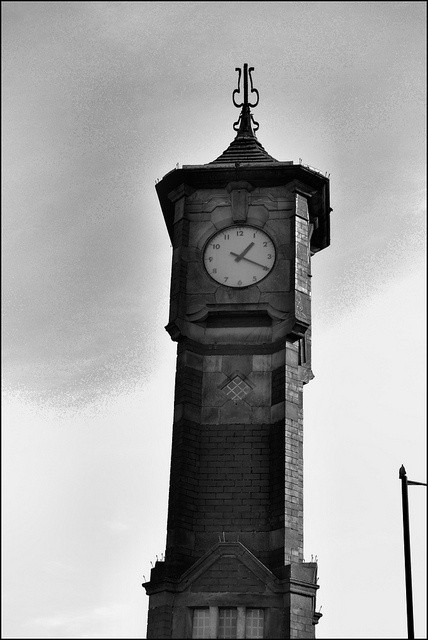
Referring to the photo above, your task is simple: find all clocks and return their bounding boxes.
[204,227,275,288]
